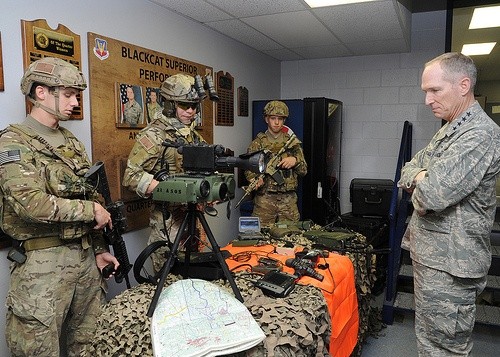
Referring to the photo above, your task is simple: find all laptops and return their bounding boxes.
[238,216,264,241]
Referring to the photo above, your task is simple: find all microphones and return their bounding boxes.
[285,258,324,282]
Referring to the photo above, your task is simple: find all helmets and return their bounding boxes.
[264,101,289,117]
[156,74,202,108]
[20,56,87,95]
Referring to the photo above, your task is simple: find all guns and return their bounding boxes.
[84,161,133,289]
[234,133,302,209]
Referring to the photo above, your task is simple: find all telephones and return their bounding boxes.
[253,271,298,298]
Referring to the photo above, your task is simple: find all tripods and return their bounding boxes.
[146,204,244,316]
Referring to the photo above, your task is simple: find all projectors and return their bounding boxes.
[151,171,237,204]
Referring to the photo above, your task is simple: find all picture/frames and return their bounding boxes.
[114,81,146,128]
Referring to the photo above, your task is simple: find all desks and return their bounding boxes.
[94,222,376,357]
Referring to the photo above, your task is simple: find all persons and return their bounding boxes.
[0,57,120,356]
[244,101,307,231]
[121,73,219,278]
[397,52,500,357]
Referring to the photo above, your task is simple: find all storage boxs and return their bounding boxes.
[340,213,391,297]
[350,177,394,218]
